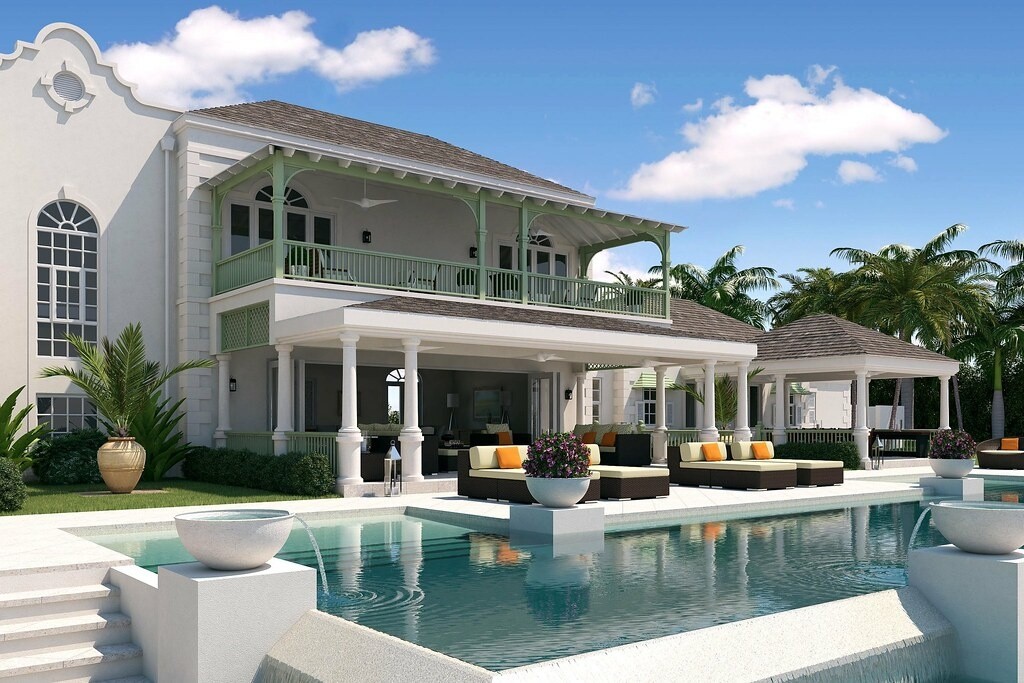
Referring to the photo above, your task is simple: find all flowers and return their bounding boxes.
[522,429,592,478]
[929,427,977,459]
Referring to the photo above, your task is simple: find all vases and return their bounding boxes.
[929,459,975,478]
[526,477,591,508]
[473,387,503,420]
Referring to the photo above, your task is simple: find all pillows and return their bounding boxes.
[702,443,722,462]
[751,442,770,459]
[601,432,617,446]
[496,448,522,469]
[496,431,512,445]
[1001,438,1019,450]
[582,431,597,444]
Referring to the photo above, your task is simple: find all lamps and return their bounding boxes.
[229,376,236,391]
[519,352,565,363]
[331,177,398,209]
[388,346,445,353]
[513,220,558,237]
[446,393,459,430]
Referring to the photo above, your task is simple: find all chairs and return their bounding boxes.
[456,269,477,299]
[489,273,536,305]
[549,289,567,308]
[573,286,596,310]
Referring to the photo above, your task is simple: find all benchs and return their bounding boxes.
[284,248,358,287]
[386,264,441,294]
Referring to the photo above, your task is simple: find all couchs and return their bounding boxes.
[976,437,1024,469]
[470,423,531,447]
[574,424,651,466]
[587,443,669,500]
[358,423,458,474]
[732,441,844,486]
[458,445,600,503]
[679,441,798,490]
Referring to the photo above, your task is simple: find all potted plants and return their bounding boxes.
[36,321,218,493]
[624,291,644,313]
